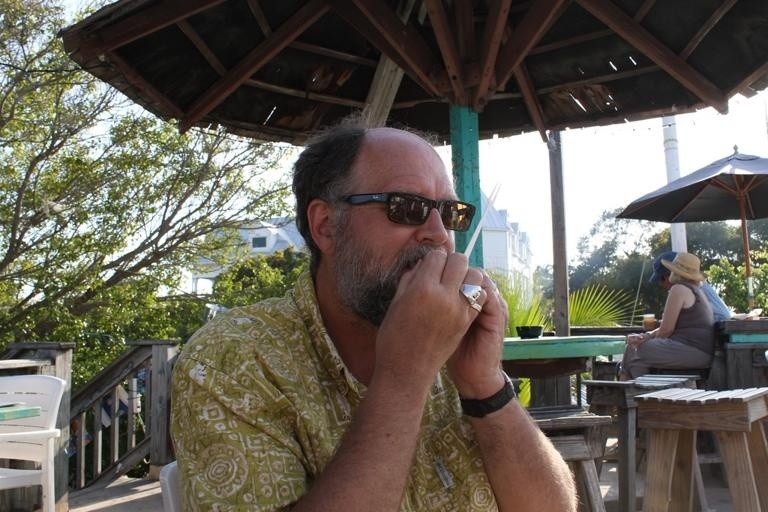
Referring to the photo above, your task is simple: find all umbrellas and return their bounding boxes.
[606,147,768,313]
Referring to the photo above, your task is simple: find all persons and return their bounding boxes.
[646,253,732,320]
[616,252,716,380]
[168,115,586,512]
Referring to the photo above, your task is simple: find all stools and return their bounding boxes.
[634,368,731,485]
[633,387,767,511]
[523,407,613,512]
[583,373,708,512]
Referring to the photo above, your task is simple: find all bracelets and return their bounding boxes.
[643,331,654,340]
[656,319,660,327]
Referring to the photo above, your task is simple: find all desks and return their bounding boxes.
[500,333,627,412]
[0,405,41,420]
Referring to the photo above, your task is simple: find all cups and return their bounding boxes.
[642,314,655,330]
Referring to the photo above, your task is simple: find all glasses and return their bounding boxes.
[337,192,477,231]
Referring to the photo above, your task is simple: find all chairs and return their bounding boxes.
[159,458,181,512]
[0,374,66,511]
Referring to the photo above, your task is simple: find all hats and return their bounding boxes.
[647,252,707,283]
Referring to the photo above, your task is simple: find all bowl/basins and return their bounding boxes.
[516,325,543,339]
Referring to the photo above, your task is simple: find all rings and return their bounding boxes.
[458,280,481,304]
[470,302,483,312]
